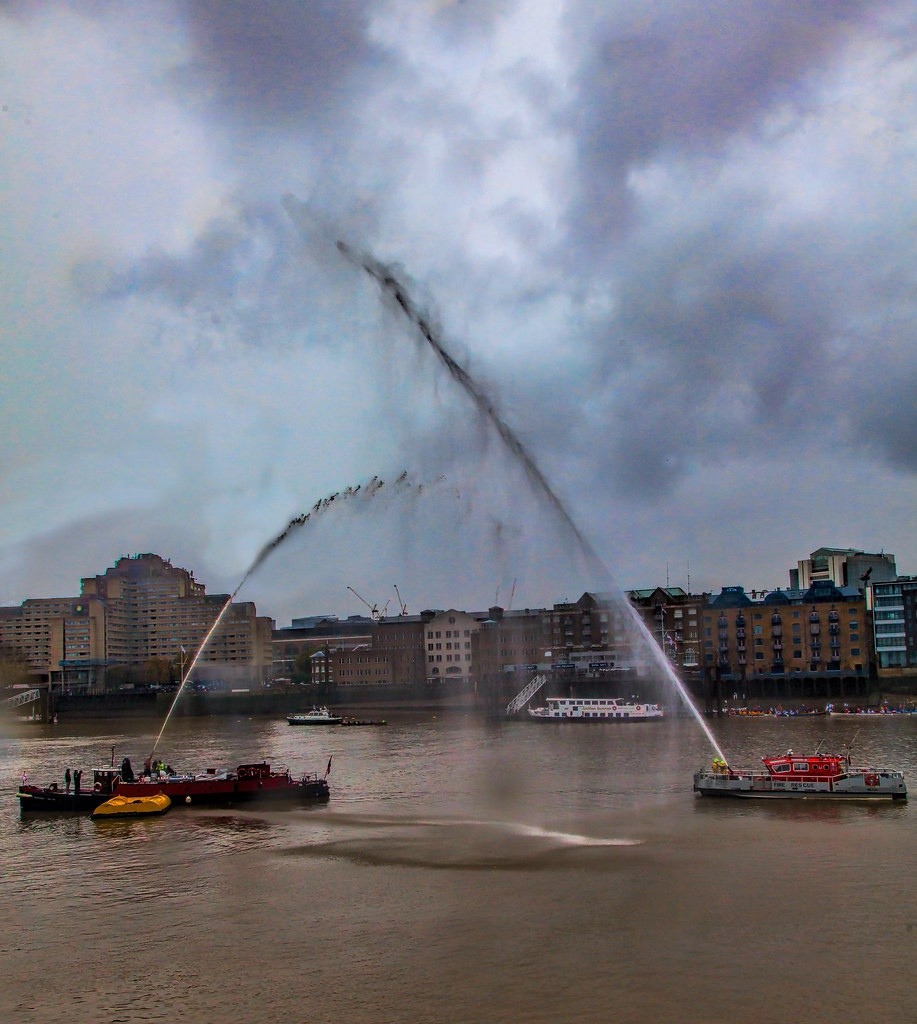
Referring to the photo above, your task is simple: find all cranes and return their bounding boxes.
[394,585,408,616]
[347,586,391,619]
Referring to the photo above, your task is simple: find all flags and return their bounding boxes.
[327,757,332,774]
[21,772,28,782]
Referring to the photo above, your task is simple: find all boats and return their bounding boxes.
[693,730,908,800]
[287,705,342,725]
[9,705,58,722]
[15,745,332,812]
[89,791,174,818]
[732,710,917,717]
[341,720,387,726]
[526,686,667,721]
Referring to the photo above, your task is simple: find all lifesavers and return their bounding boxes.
[636,706,641,710]
[864,774,879,786]
[613,706,617,711]
[93,782,103,792]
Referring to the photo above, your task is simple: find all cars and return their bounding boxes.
[160,684,211,692]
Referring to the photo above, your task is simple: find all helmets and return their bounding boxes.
[721,761,725,764]
[714,759,719,762]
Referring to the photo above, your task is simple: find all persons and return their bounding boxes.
[65,756,174,796]
[711,758,728,774]
